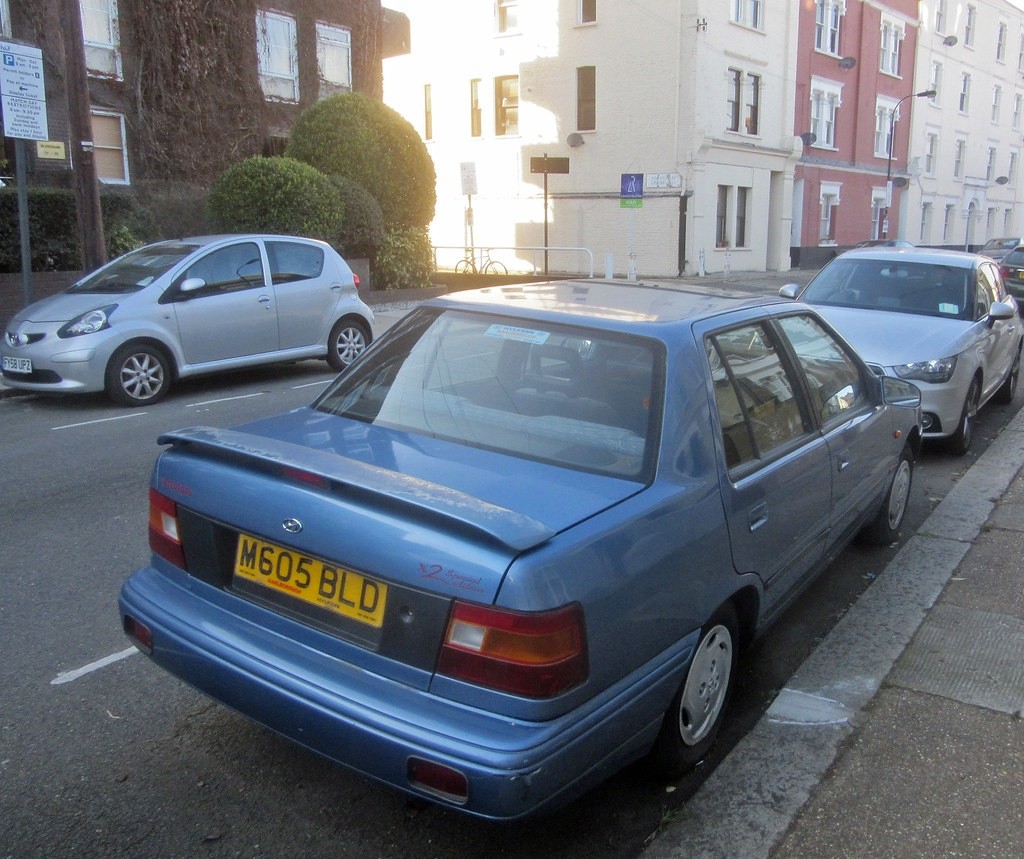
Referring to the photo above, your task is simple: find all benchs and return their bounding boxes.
[474,381,624,429]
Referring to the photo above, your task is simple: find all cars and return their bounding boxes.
[0,233,378,407]
[976,238,1024,265]
[856,240,914,250]
[117,276,922,826]
[778,244,1023,460]
[998,244,1023,315]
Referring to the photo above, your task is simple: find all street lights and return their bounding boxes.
[881,90,935,239]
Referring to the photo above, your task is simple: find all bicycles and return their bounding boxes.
[454,246,508,275]
